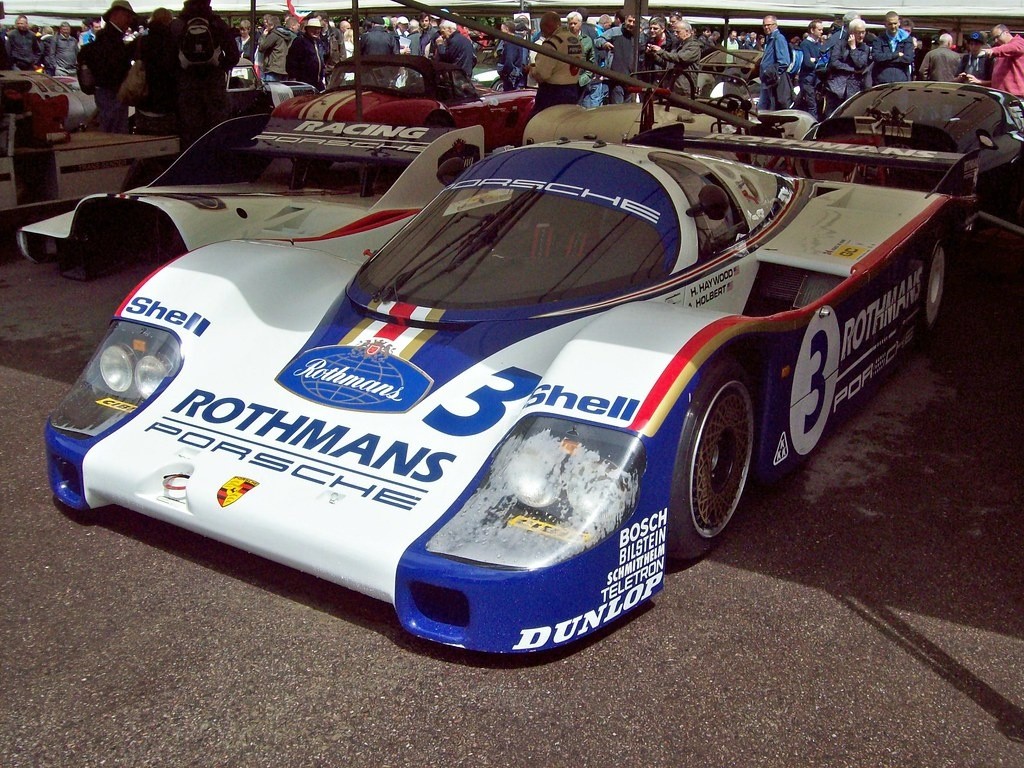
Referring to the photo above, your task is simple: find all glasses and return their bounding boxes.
[989,31,1004,41]
[851,31,866,35]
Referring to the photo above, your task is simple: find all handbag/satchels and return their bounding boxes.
[117,60,150,113]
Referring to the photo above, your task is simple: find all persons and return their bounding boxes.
[521,10,584,126]
[123,17,149,43]
[493,14,546,92]
[90,0,135,133]
[125,7,177,136]
[789,10,992,121]
[232,8,474,95]
[562,7,764,109]
[0,13,102,78]
[759,14,793,110]
[164,0,241,155]
[981,22,1024,107]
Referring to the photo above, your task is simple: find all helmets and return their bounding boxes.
[671,11,682,21]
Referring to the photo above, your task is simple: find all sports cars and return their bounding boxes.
[45,121,983,659]
[1,50,1024,282]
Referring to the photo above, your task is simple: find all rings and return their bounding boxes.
[988,52,990,53]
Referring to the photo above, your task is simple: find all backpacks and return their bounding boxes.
[178,9,220,80]
[772,32,804,74]
[77,35,121,96]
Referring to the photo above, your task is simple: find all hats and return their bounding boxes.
[102,0,140,23]
[305,18,324,28]
[370,17,385,24]
[515,23,529,32]
[430,9,450,19]
[968,32,985,44]
[383,17,390,27]
[396,16,410,24]
